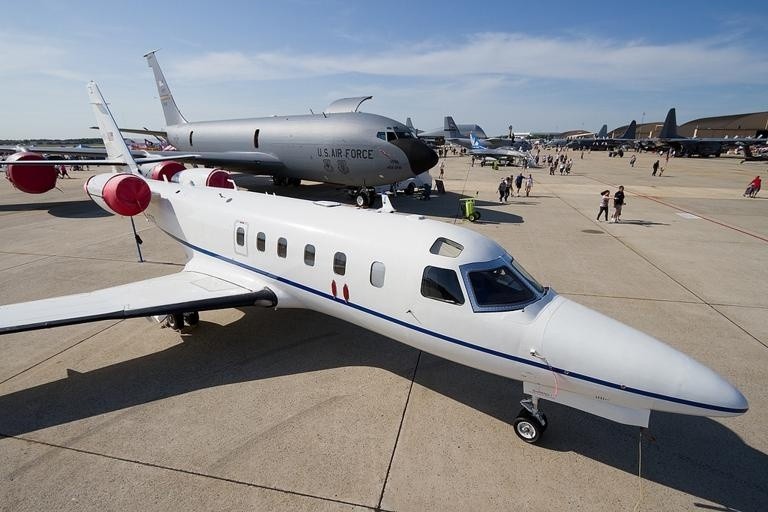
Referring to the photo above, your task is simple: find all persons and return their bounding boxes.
[596,190,619,223]
[55,164,63,179]
[626,143,676,176]
[749,177,761,197]
[472,144,591,203]
[438,161,447,178]
[617,202,626,220]
[438,142,466,158]
[611,186,626,222]
[59,165,71,179]
